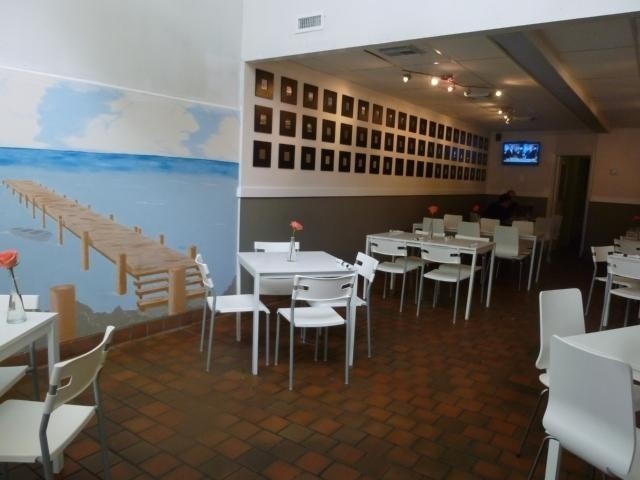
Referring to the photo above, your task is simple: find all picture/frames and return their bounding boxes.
[250,66,518,184]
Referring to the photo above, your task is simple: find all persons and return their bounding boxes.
[485,194,512,220]
[419,201,443,222]
[462,200,481,223]
[505,143,538,159]
[507,189,522,217]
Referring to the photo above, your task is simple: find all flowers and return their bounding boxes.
[289,220,303,236]
[0,250,21,297]
[428,206,439,215]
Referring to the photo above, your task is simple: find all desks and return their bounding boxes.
[413,223,545,291]
[235,250,359,376]
[362,231,496,321]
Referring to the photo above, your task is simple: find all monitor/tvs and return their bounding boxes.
[501,141,541,165]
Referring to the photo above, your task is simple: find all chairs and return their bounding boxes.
[300,252,380,360]
[436,235,490,304]
[0,294,40,400]
[525,335,639,480]
[369,237,418,304]
[493,225,528,289]
[584,230,640,332]
[275,273,356,389]
[516,284,590,460]
[0,325,117,479]
[395,230,446,297]
[194,253,271,373]
[421,206,561,240]
[416,241,473,324]
[512,220,534,257]
[254,240,307,344]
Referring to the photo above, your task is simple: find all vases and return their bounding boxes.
[7,278,26,324]
[427,220,434,240]
[287,237,297,262]
